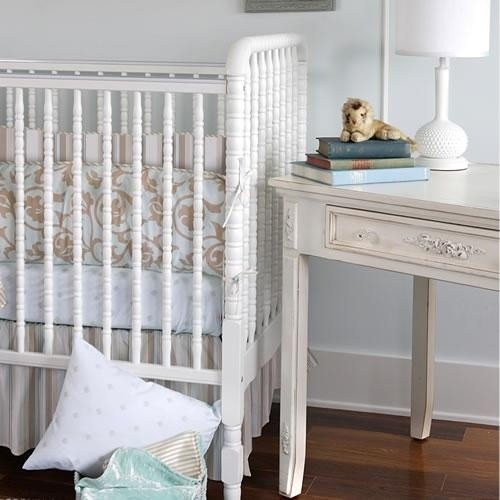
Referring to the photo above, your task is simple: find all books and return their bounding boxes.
[316,136,411,159]
[289,161,429,187]
[306,152,415,169]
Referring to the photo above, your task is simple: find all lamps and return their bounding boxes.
[390,0,495,172]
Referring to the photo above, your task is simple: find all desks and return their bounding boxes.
[267,154,498,499]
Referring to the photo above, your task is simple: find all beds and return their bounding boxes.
[3,34,306,500]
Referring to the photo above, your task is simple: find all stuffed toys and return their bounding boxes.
[339,97,415,144]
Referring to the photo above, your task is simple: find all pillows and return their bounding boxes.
[23,335,224,475]
